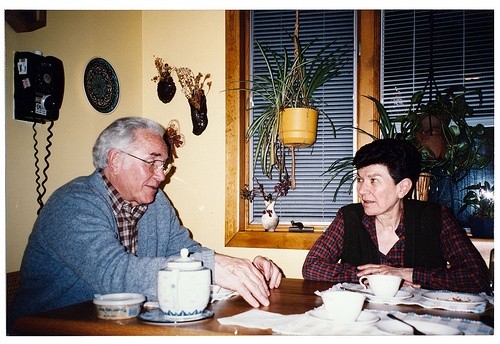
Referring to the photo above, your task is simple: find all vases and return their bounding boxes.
[261,200,279,233]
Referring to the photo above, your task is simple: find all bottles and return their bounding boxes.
[157,248,211,320]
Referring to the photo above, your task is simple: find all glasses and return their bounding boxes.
[117,149,172,175]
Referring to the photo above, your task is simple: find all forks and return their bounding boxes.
[387,313,427,335]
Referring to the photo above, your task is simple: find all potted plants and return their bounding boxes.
[317,84,465,204]
[396,84,491,184]
[445,181,494,239]
[215,23,357,185]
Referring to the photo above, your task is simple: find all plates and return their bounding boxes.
[210,284,239,304]
[421,291,486,307]
[138,309,215,326]
[372,320,464,335]
[306,310,380,326]
[360,289,413,301]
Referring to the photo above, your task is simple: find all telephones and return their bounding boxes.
[12,50,65,125]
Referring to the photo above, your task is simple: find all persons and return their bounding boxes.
[10,117,282,316]
[302,139,490,293]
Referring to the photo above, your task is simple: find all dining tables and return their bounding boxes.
[10,277,494,336]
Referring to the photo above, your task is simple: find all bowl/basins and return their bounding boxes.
[93,292,146,320]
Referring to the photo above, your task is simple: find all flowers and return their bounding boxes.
[239,175,292,217]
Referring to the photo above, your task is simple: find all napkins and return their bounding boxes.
[477,291,494,306]
[272,313,333,336]
[313,282,487,314]
[305,307,494,335]
[216,311,294,330]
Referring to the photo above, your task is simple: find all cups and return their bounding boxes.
[320,291,366,321]
[359,275,402,297]
